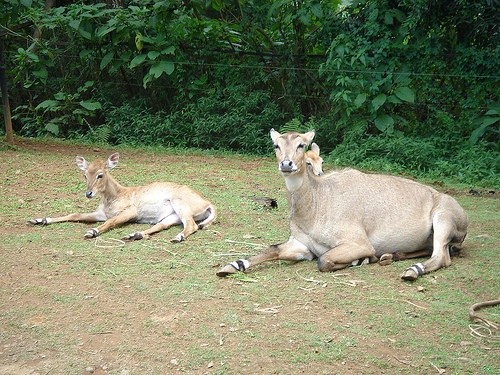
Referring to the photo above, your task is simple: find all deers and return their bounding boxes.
[26,152,217,243]
[216,128,467,281]
